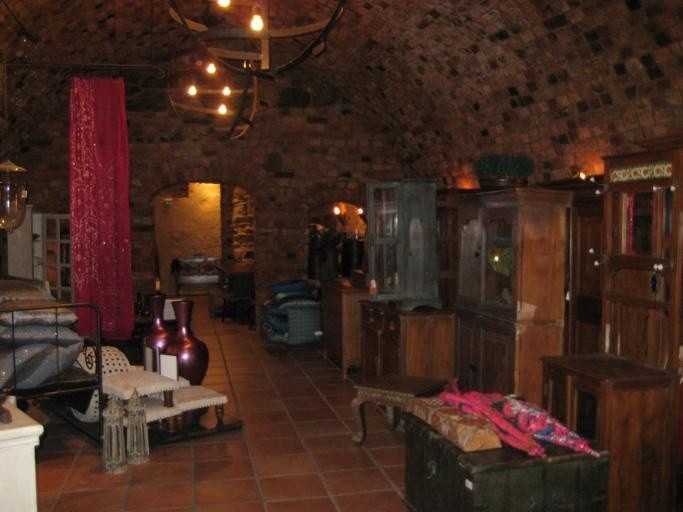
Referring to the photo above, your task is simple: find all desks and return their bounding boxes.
[171,257,254,329]
[0,401,44,512]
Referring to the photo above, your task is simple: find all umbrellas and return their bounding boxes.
[466,390,602,457]
[428,377,553,465]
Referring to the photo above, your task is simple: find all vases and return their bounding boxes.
[164,301,209,386]
[139,293,173,354]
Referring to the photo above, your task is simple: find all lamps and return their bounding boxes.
[0,160,28,233]
[165,47,260,139]
[167,0,346,85]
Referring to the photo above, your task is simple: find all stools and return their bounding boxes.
[102,371,228,435]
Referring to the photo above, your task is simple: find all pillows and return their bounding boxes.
[0,276,87,389]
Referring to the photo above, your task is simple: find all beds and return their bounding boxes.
[0,275,103,449]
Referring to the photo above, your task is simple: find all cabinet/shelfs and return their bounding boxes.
[321,146,683,511]
[7,204,71,302]
